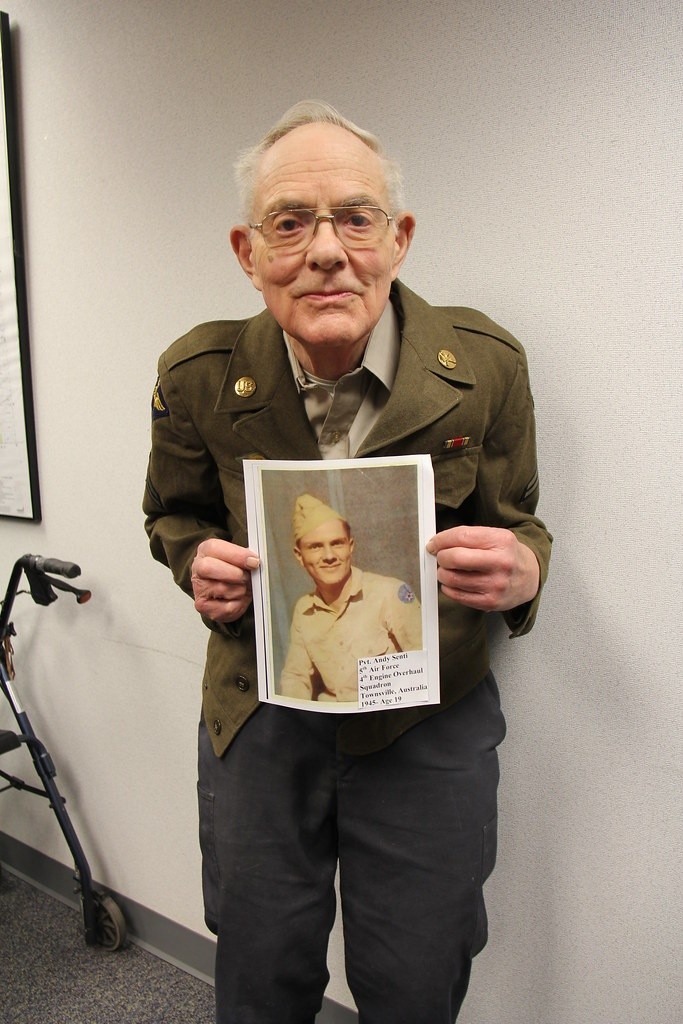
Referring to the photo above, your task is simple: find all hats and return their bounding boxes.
[293,494,347,543]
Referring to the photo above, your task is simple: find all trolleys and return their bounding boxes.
[0,554,130,953]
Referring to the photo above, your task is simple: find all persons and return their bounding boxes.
[143,99,552,1024]
[279,494,423,701]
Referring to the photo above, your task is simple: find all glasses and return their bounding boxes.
[250,206,394,255]
